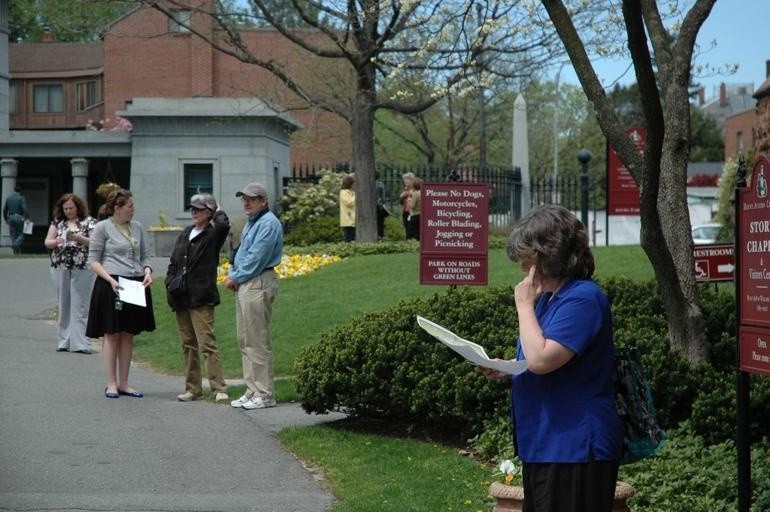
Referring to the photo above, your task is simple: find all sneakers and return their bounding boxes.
[56,348,67,352]
[230,395,248,408]
[215,392,228,402]
[241,396,276,409]
[178,392,204,401]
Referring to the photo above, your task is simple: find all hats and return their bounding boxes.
[184,193,217,212]
[235,183,266,198]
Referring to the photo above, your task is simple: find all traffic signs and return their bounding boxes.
[691,243,739,283]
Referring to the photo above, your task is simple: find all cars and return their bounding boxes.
[691,222,723,243]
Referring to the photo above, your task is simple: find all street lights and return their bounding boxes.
[474,1,488,164]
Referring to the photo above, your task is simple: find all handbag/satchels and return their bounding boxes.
[611,344,664,466]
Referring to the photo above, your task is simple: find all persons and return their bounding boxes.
[44,193,100,354]
[472,203,625,512]
[85,188,153,399]
[165,193,232,402]
[224,182,284,410]
[3,186,31,254]
[335,168,424,242]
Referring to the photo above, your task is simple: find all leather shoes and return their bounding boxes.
[106,392,119,398]
[118,388,143,397]
